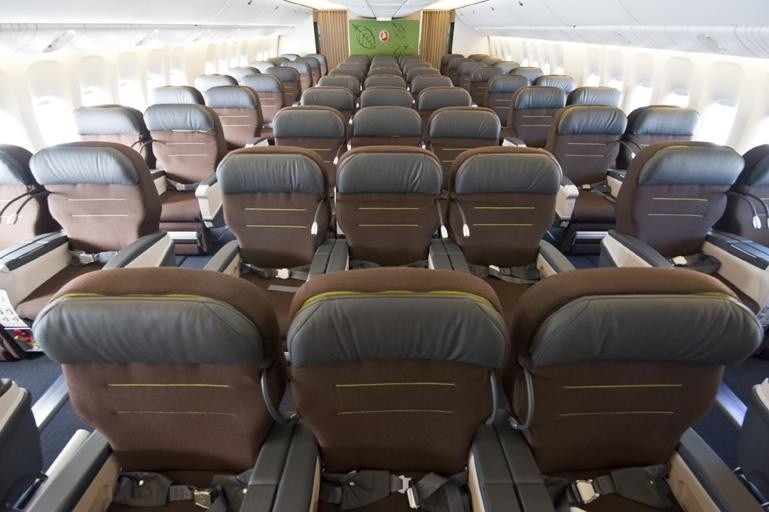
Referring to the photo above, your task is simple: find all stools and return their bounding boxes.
[732,370,769,500]
[0,376,44,511]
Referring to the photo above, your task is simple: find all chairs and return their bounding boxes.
[31,265,298,511]
[0,52,769,324]
[488,264,764,510]
[267,265,524,511]
